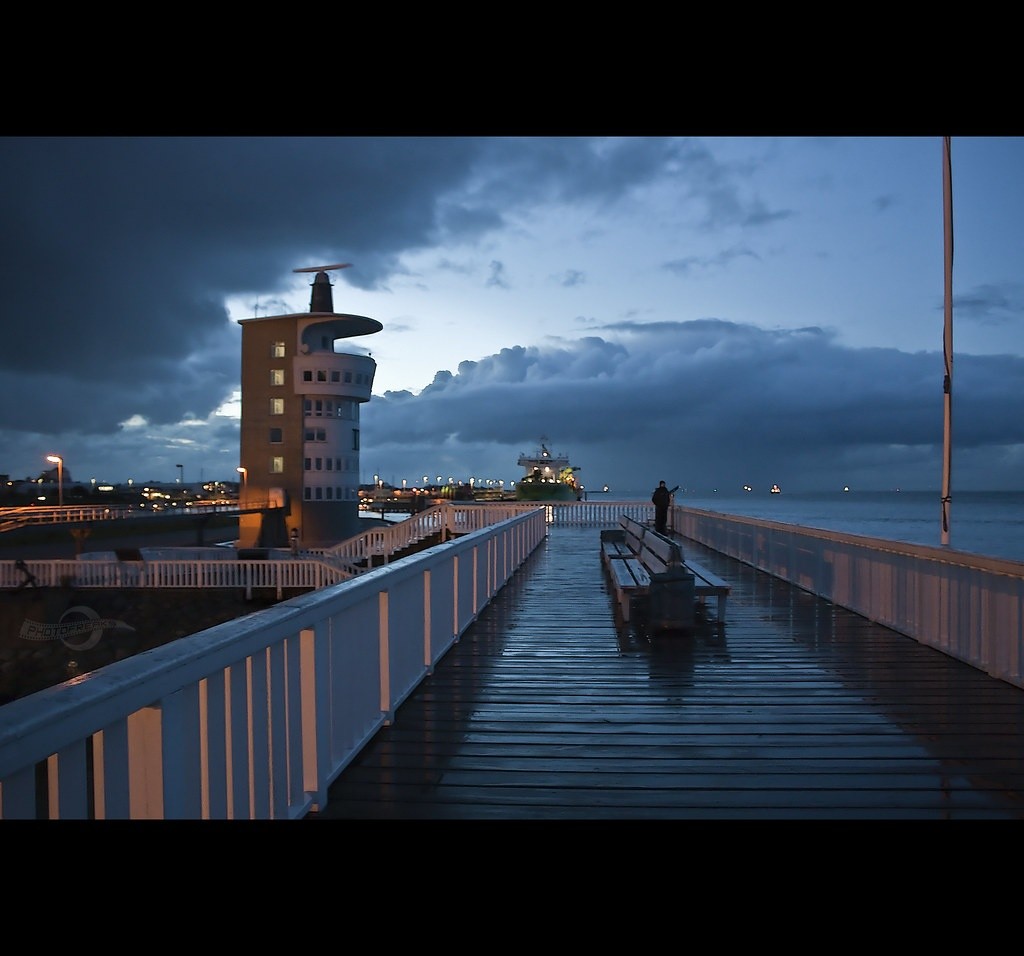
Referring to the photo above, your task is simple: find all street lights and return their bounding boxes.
[46,456,64,507]
[176,463,184,509]
[235,468,248,510]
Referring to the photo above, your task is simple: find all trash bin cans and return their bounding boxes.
[649,572,697,631]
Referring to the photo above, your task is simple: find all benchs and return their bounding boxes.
[600,514,732,624]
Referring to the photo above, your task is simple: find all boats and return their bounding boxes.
[514,437,585,503]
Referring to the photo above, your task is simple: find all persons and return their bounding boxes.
[651,481,670,538]
